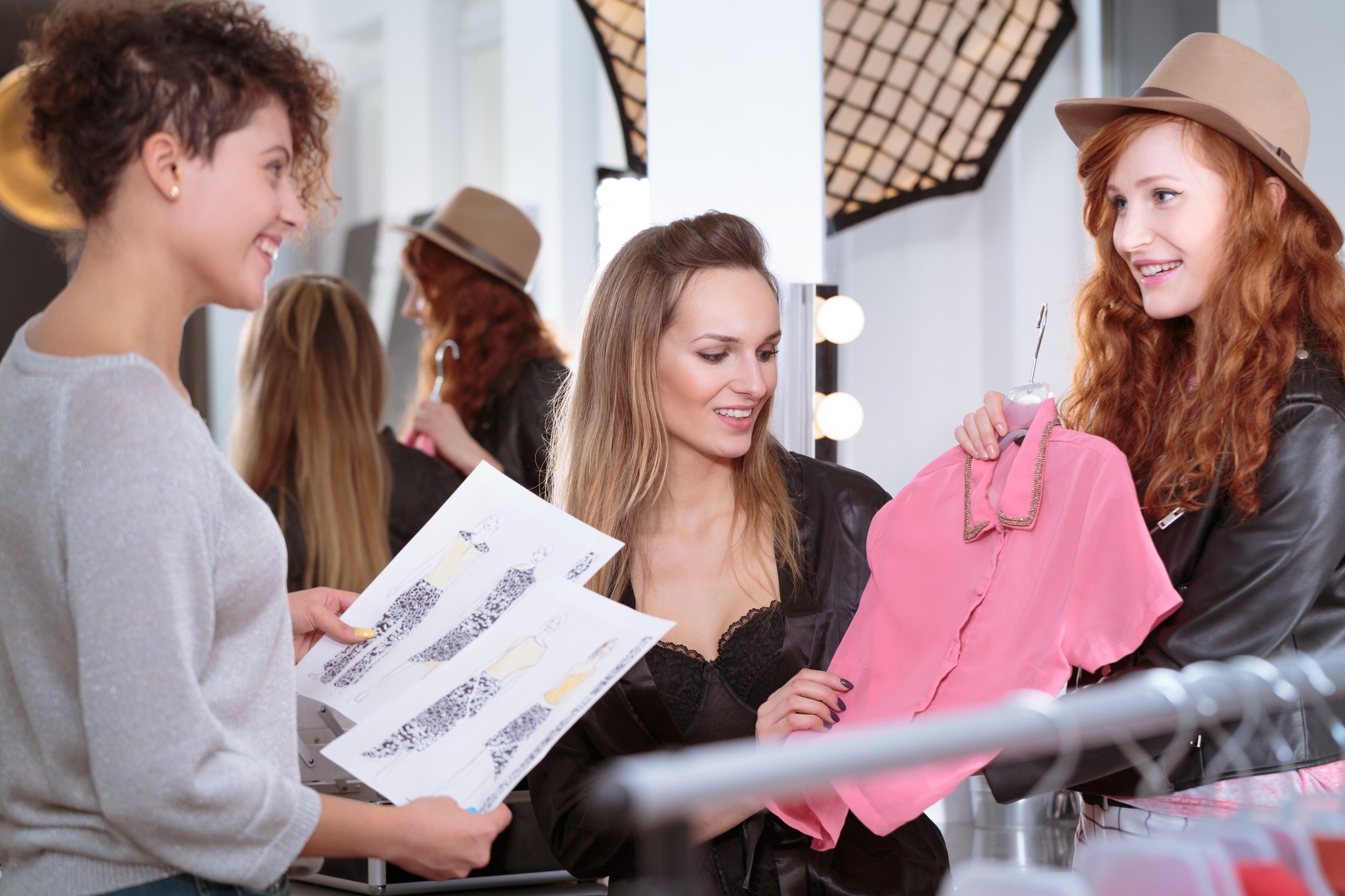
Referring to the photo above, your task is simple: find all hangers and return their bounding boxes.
[913,304,1122,478]
[429,339,459,401]
[942,650,1345,896]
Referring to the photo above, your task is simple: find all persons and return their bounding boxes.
[0,0,513,896]
[225,273,462,591]
[954,31,1345,843]
[389,188,572,500]
[529,213,950,896]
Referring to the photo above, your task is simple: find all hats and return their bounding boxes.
[393,186,543,293]
[1055,32,1344,254]
